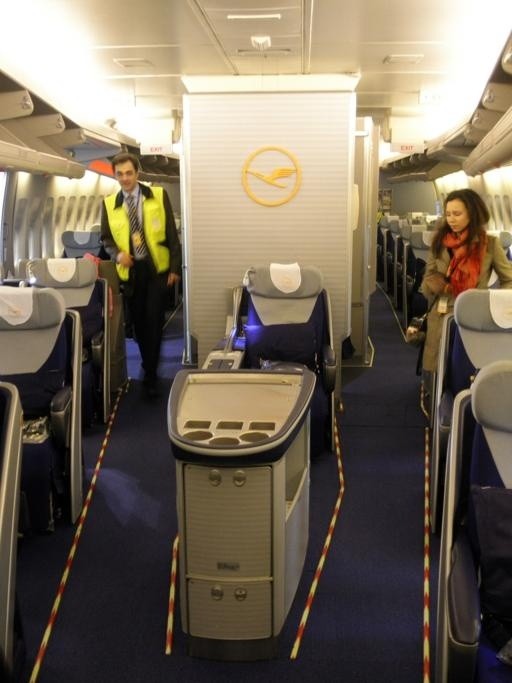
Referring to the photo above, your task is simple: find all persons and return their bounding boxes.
[421,187,512,374]
[99,151,182,401]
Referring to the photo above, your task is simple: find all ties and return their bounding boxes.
[126,194,148,261]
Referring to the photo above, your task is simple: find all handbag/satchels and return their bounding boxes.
[405,313,426,346]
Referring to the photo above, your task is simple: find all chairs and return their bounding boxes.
[201,263,338,453]
